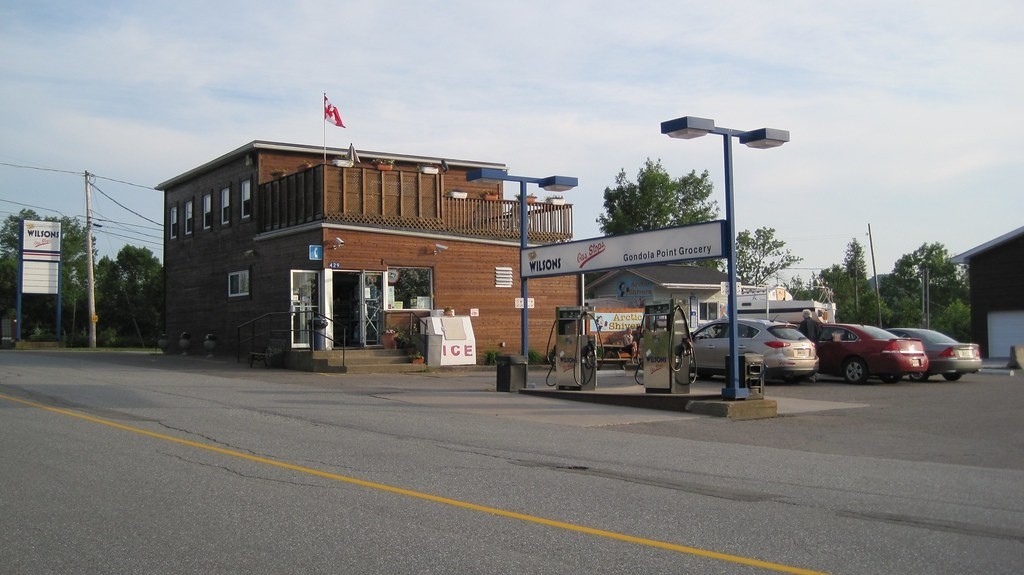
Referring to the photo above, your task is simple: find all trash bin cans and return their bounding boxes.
[308,318,328,351]
[494,353,528,392]
[725,353,765,400]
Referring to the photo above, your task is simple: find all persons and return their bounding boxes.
[633,325,644,344]
[799,309,823,383]
[622,327,638,364]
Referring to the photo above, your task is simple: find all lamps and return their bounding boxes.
[818,310,828,320]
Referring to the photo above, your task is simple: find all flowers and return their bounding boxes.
[160,332,215,341]
[443,306,452,311]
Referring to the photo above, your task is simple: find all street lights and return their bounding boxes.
[464,165,581,361]
[661,115,791,402]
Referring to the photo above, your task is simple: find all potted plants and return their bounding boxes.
[380,319,424,364]
[269,155,565,205]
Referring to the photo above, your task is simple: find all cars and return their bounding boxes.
[881,326,980,382]
[689,316,819,385]
[805,321,928,385]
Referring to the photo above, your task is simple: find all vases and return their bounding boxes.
[158,339,217,360]
[444,311,452,316]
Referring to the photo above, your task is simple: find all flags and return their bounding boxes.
[324,96,346,129]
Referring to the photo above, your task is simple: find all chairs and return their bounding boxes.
[820,328,834,341]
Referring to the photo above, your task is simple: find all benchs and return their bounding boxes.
[596,344,641,371]
